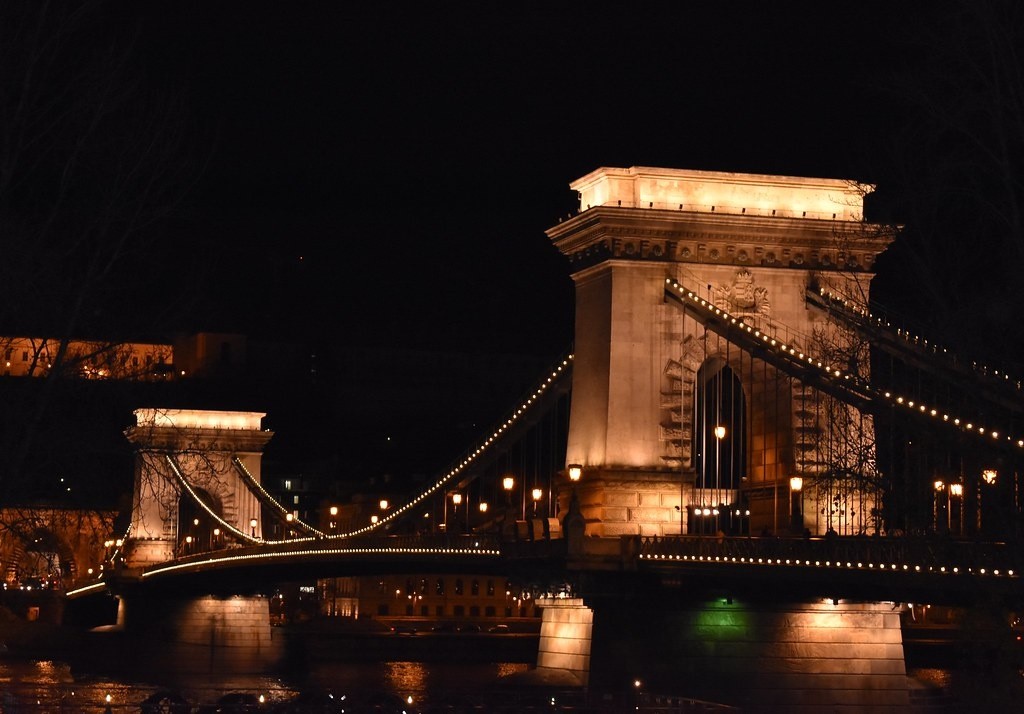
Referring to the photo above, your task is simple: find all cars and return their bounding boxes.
[384,622,512,632]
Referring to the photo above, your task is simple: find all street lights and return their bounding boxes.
[249,516,257,537]
[745,510,753,537]
[951,483,963,536]
[531,488,542,516]
[503,476,514,511]
[480,502,489,532]
[715,425,726,504]
[703,509,710,532]
[568,463,582,506]
[735,510,742,534]
[790,476,803,535]
[104,541,110,561]
[213,528,221,551]
[379,501,387,541]
[695,509,702,534]
[330,505,338,535]
[186,536,193,555]
[285,512,293,539]
[933,481,944,534]
[453,492,462,517]
[193,519,199,550]
[713,510,720,534]
